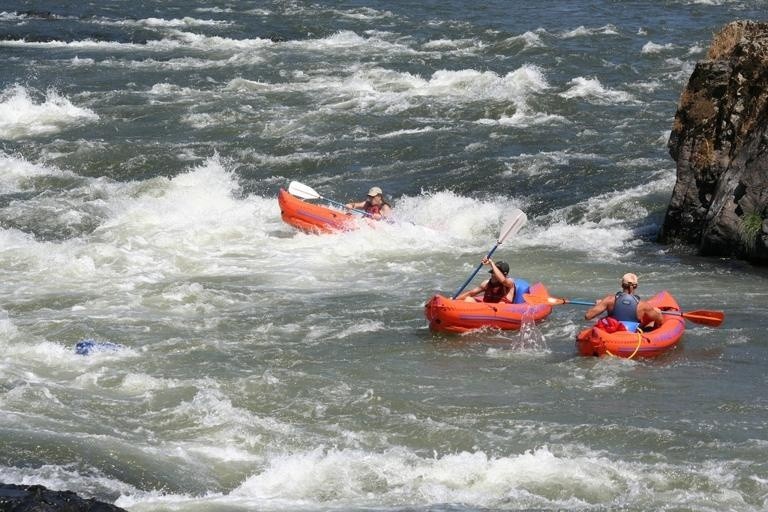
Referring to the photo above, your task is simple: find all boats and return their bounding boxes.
[425,282,553,337]
[577,289,686,358]
[278,186,393,236]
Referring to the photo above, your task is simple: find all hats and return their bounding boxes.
[489,261,508,273]
[368,187,382,196]
[622,273,637,284]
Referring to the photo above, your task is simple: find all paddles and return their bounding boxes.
[288,180,373,216]
[453,208,527,298]
[522,293,724,327]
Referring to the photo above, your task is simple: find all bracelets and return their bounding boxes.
[350,203,355,209]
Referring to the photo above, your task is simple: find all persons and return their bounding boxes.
[450,255,517,304]
[345,186,392,222]
[584,272,662,332]
